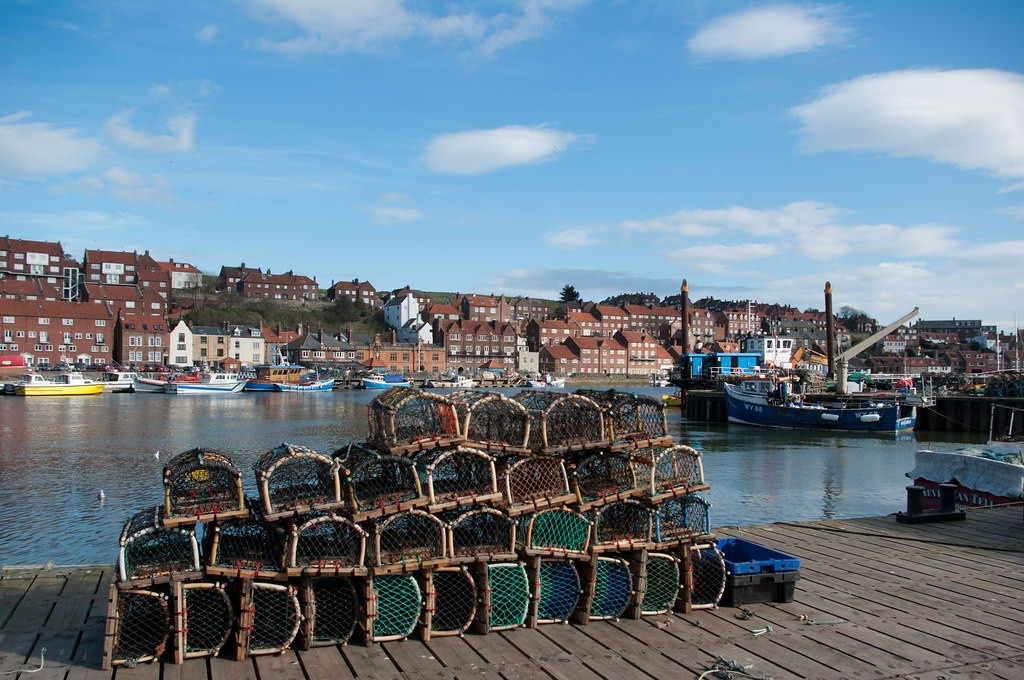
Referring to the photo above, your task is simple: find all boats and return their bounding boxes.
[722,378,917,435]
[133,372,249,394]
[661,394,681,406]
[242,364,333,391]
[0,370,139,396]
[361,375,412,389]
[649,376,672,388]
[164,378,248,393]
[274,378,334,392]
[527,377,564,389]
[913,403,1024,511]
[430,376,472,388]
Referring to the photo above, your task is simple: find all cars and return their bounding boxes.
[34,363,224,372]
